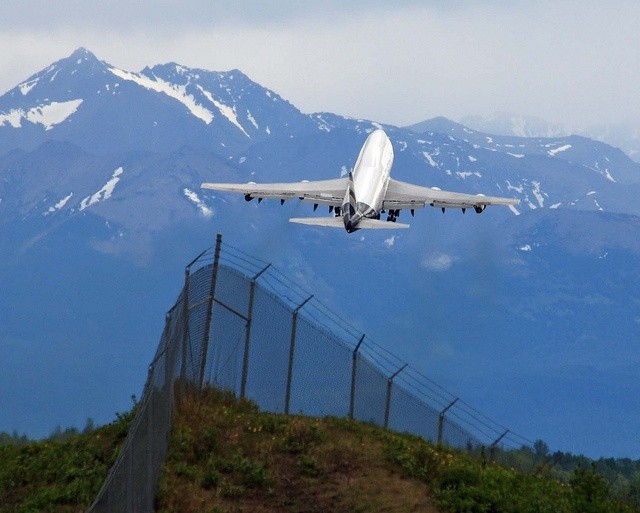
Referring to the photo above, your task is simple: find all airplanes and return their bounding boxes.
[200,128,521,233]
